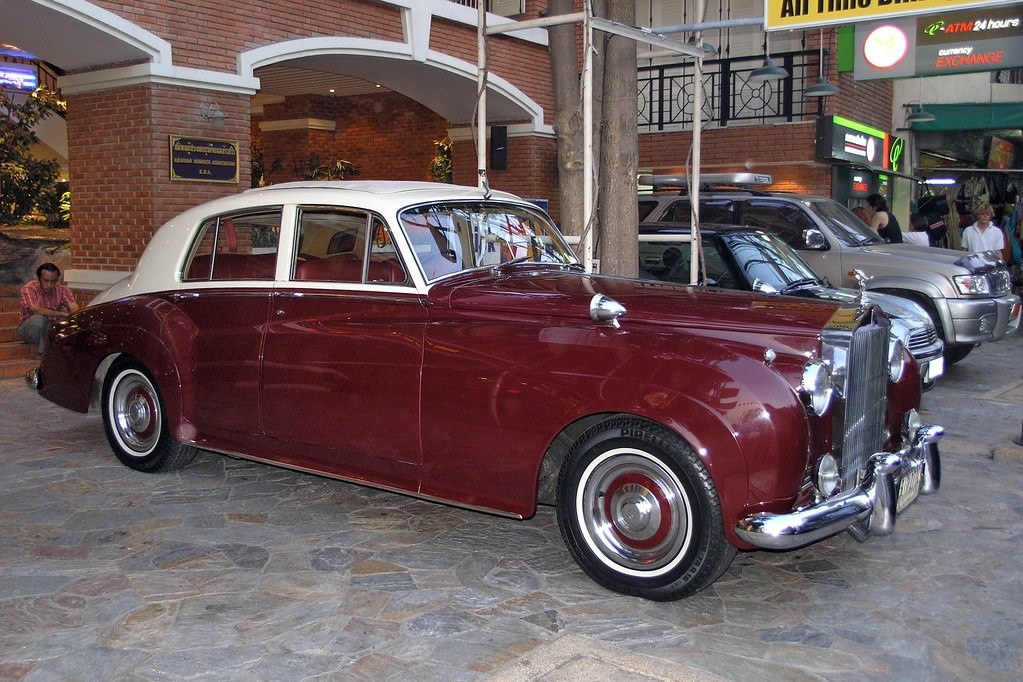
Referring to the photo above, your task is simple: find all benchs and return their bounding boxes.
[186,252,323,281]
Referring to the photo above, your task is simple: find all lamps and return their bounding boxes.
[906,75,936,121]
[803,26,839,95]
[201,101,231,128]
[748,32,789,80]
[674,26,718,57]
[925,170,955,185]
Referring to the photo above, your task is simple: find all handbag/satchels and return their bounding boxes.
[918,182,949,217]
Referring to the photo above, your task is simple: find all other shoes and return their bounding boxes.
[35,353,42,359]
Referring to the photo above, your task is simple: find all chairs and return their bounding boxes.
[297,259,390,284]
[660,247,690,283]
[382,255,455,284]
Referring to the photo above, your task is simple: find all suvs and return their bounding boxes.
[638,173,1021,365]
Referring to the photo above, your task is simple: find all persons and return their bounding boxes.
[18,263,80,361]
[866,193,903,244]
[961,202,1004,261]
[900,213,929,249]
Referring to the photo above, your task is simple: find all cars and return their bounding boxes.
[638,220,946,393]
[23,178,944,604]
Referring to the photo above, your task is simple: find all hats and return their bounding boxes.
[973,202,995,217]
